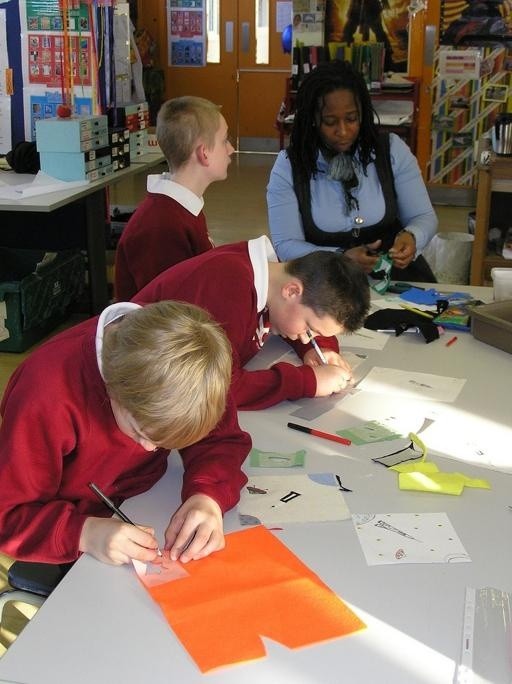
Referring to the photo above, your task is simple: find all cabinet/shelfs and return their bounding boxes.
[275,71,424,164]
[467,133,512,285]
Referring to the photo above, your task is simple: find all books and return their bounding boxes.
[328,43,385,92]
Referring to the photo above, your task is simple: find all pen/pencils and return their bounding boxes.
[287,423,351,446]
[306,330,328,364]
[87,481,163,557]
[446,336,457,346]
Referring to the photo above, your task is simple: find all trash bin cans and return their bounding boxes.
[434,232,475,285]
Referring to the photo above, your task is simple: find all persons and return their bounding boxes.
[0,299,252,565]
[114,98,235,301]
[130,235,371,411]
[265,59,440,282]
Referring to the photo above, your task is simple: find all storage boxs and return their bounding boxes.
[1,245,90,354]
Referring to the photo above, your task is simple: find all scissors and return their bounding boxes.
[389,283,458,296]
[361,243,384,258]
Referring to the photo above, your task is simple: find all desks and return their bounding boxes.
[134,152,166,167]
[1,162,146,322]
[2,278,511,683]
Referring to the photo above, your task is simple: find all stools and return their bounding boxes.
[1,551,79,654]
